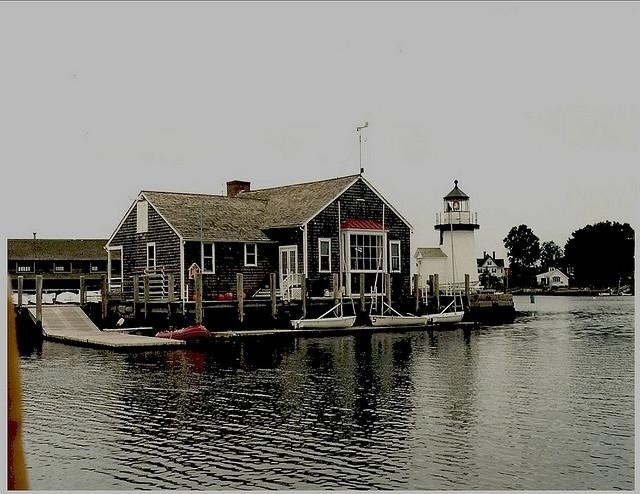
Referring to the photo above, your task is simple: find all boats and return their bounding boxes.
[598,287,634,298]
[166,345,207,373]
[154,324,212,342]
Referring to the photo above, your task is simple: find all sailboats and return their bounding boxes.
[282,336,355,412]
[372,331,429,398]
[435,329,466,412]
[290,201,357,329]
[369,203,428,325]
[421,210,465,323]
